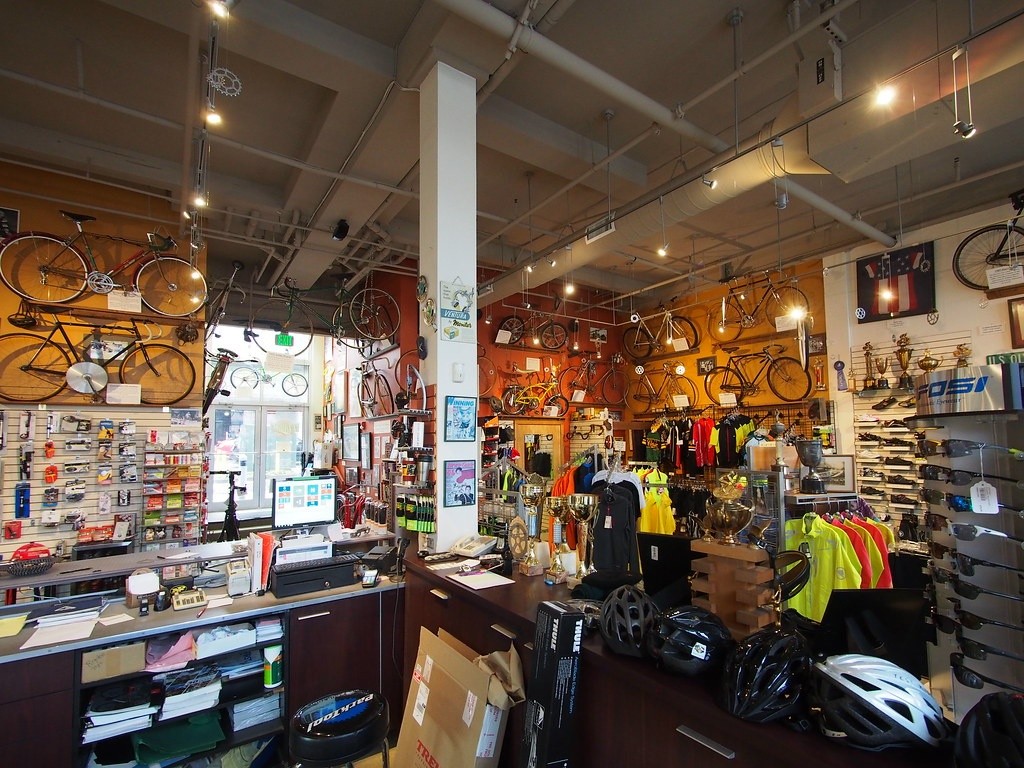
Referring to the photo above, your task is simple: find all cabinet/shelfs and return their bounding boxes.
[140,449,204,551]
[395,408,434,499]
[853,421,925,508]
[0,572,406,768]
[402,556,959,768]
[478,415,501,497]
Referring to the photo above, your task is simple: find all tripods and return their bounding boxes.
[208,471,247,543]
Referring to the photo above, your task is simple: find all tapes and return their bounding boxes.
[141,599,148,611]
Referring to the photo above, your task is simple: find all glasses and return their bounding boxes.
[917,438,1024,693]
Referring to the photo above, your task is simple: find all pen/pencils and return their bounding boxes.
[204,567,219,572]
[59,568,91,574]
[196,608,205,618]
[157,555,165,559]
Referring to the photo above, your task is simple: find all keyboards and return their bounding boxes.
[271,554,359,573]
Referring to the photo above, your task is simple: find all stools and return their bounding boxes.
[290,690,394,768]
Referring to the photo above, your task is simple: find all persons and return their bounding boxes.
[454,485,473,504]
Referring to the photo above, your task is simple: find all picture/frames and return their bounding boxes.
[696,356,717,377]
[444,461,477,508]
[809,332,827,357]
[798,454,855,493]
[444,396,479,441]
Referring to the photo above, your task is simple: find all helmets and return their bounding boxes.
[809,652,950,751]
[600,584,661,658]
[951,691,1024,768]
[716,621,814,721]
[640,603,737,689]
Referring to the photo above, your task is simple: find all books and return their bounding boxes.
[227,693,280,732]
[26,597,102,628]
[82,660,222,736]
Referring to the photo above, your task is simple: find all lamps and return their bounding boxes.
[486,297,493,326]
[547,257,556,267]
[702,174,718,187]
[770,139,790,210]
[532,316,538,347]
[658,196,670,256]
[954,43,977,140]
[528,265,537,272]
[522,270,533,309]
[183,0,240,306]
[332,219,350,240]
[573,276,750,360]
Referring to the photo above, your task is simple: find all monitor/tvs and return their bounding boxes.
[271,475,336,536]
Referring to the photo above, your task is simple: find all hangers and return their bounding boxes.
[791,501,882,535]
[556,448,667,494]
[653,403,751,424]
[508,462,528,482]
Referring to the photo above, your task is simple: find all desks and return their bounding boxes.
[0,522,397,608]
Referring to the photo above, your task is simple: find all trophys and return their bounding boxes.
[795,440,827,494]
[518,484,543,576]
[545,497,570,584]
[862,342,878,390]
[769,409,795,491]
[917,349,944,374]
[874,356,890,389]
[954,344,970,368]
[567,493,598,590]
[893,334,914,387]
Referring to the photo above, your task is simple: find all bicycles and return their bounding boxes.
[623,296,701,360]
[1,306,196,404]
[231,359,310,397]
[355,358,394,419]
[705,267,811,344]
[705,345,813,409]
[495,290,570,350]
[500,371,569,417]
[951,186,1023,291]
[559,350,631,405]
[203,348,238,416]
[623,359,699,416]
[1,210,210,317]
[203,259,402,358]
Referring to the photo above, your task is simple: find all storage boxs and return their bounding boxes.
[81,639,146,685]
[192,624,259,660]
[392,625,519,768]
[521,600,586,768]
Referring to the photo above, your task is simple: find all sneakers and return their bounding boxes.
[852,398,926,522]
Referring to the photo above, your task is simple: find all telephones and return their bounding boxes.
[450,532,498,557]
